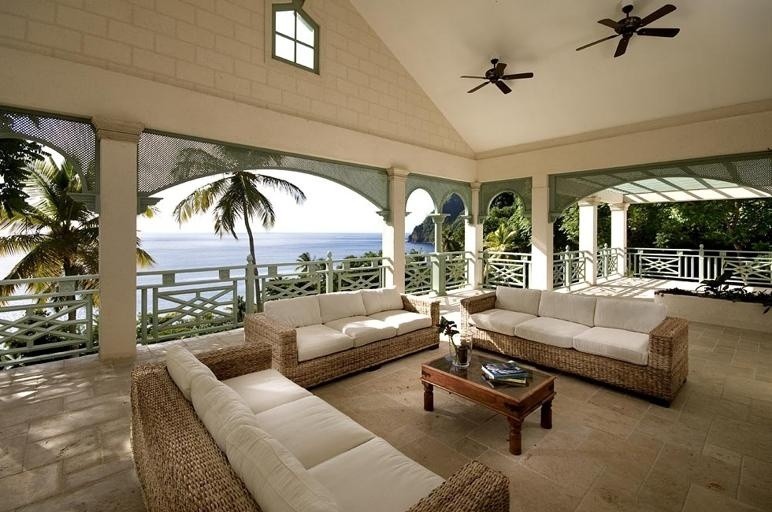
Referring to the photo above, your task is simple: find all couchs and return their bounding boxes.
[131,340,509,511]
[245,284,441,388]
[460,286,689,406]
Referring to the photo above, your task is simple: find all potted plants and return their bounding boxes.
[435,316,472,368]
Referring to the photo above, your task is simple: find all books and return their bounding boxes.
[481,362,533,380]
[488,374,527,387]
[482,374,515,389]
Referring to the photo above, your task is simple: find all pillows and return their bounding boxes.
[494,285,667,336]
[263,285,404,329]
[165,343,341,512]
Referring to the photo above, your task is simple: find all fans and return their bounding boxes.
[460,58,533,94]
[576,1,680,57]
